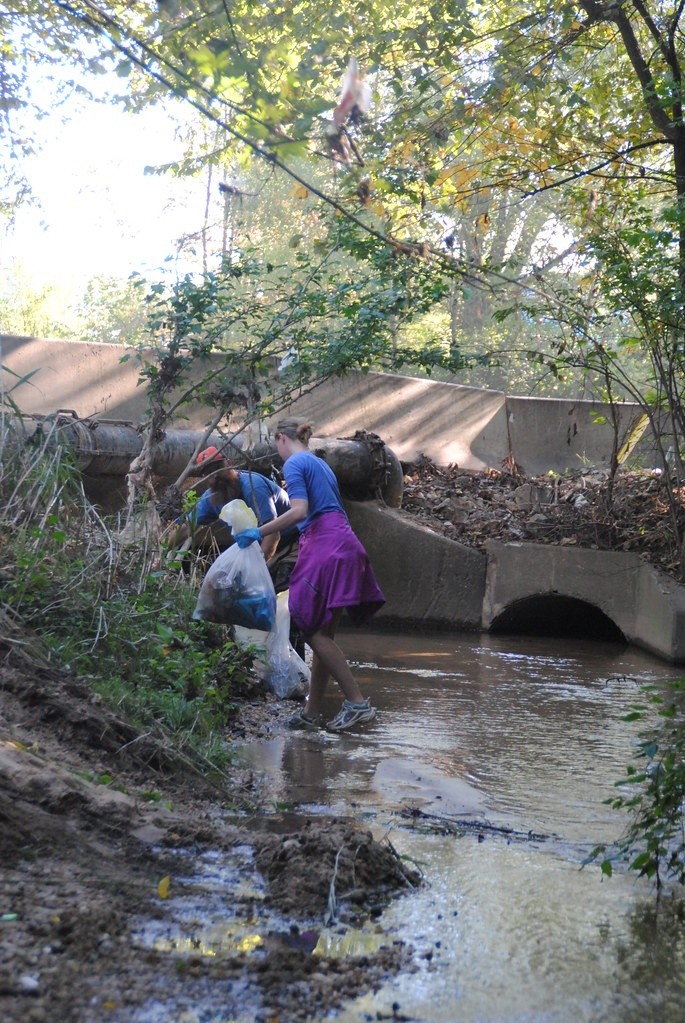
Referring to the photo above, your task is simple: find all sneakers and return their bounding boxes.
[327,696,376,730]
[295,710,316,726]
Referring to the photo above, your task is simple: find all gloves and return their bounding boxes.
[235,528,261,549]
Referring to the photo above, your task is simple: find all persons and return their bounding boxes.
[234,416,386,730]
[168,446,299,594]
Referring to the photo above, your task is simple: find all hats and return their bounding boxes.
[195,447,227,475]
